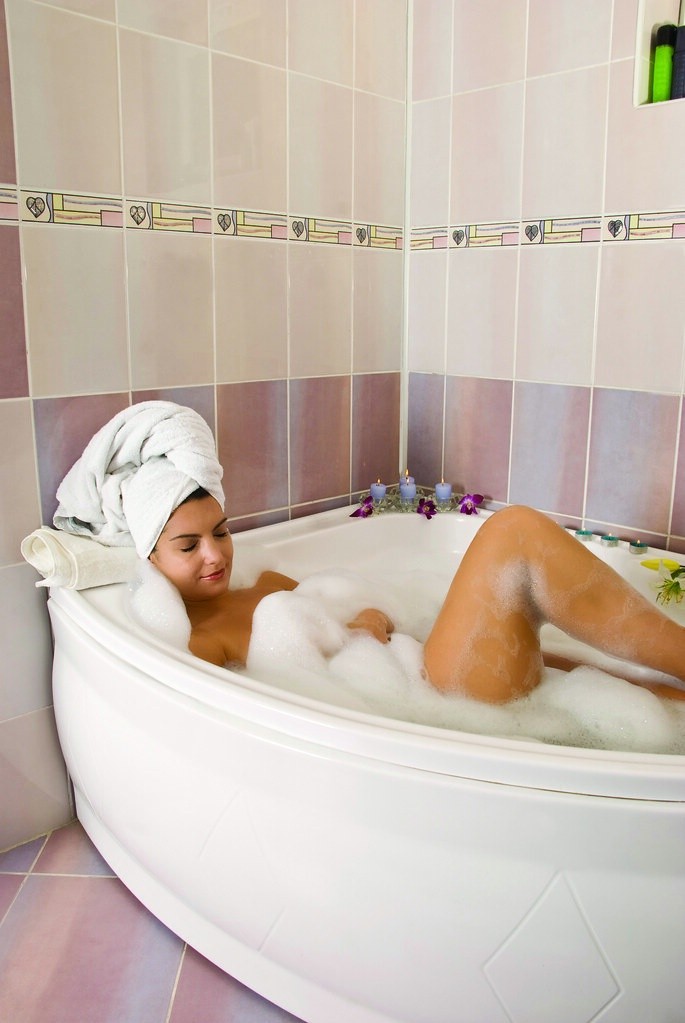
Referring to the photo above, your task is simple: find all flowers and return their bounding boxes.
[349,495,374,518]
[640,558,685,606]
[417,498,437,520]
[458,494,484,515]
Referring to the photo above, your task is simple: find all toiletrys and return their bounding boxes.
[672,26,685,99]
[652,24,677,103]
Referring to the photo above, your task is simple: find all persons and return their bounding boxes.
[51,401,685,707]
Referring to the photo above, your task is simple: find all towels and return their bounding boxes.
[19,529,141,592]
[52,399,226,561]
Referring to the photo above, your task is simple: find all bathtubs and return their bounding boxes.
[45,493,685,1023]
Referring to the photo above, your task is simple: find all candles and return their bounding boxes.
[436,478,451,503]
[370,479,386,498]
[629,540,647,554]
[399,470,416,503]
[575,528,592,541]
[601,533,618,548]
